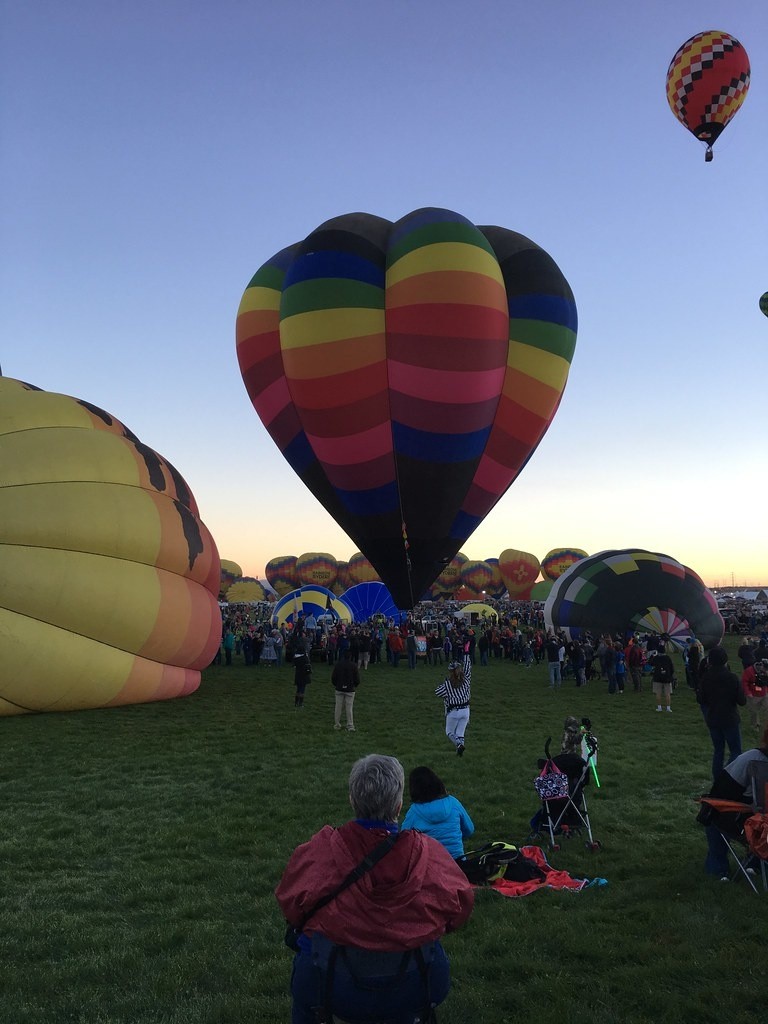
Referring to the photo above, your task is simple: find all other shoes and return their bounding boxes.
[348,728,355,731]
[334,727,340,729]
[656,709,662,712]
[457,743,465,757]
[666,710,672,712]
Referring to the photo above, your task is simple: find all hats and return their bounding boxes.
[709,647,728,665]
[448,661,461,670]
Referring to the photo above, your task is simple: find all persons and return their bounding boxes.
[436,642,473,759]
[558,716,587,755]
[214,600,768,713]
[330,651,359,731]
[273,752,475,1024]
[292,646,313,707]
[695,649,746,774]
[742,659,768,731]
[696,745,768,883]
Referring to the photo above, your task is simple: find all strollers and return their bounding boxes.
[525,734,605,857]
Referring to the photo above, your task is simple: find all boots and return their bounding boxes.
[299,694,304,707]
[295,693,300,707]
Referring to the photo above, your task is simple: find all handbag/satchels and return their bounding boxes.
[304,664,312,675]
[284,927,303,952]
[533,759,569,800]
[640,650,647,665]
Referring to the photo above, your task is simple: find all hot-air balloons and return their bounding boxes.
[237,207,578,660]
[665,30,752,159]
[2,374,725,716]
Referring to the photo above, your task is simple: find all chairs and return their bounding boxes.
[701,761,768,894]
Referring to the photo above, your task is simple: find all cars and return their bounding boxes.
[417,616,452,632]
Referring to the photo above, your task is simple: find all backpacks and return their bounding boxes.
[337,662,354,692]
[653,664,670,683]
[456,841,547,886]
